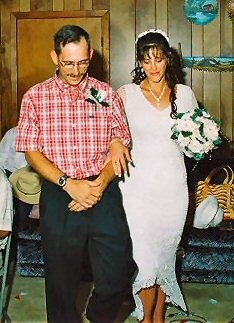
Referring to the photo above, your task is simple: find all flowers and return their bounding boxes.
[85,86,110,107]
[170,107,223,160]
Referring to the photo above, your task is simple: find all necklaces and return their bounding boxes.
[148,83,167,104]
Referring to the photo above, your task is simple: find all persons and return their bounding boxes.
[0,167,14,298]
[15,24,134,323]
[108,28,199,323]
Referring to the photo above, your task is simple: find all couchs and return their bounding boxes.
[14,101,234,285]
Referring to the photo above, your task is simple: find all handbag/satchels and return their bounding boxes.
[188,163,234,219]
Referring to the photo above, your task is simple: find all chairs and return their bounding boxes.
[0,165,14,323]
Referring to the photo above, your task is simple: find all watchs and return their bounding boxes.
[57,174,69,189]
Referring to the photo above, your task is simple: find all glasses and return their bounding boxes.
[57,54,91,68]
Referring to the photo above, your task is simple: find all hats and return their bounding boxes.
[8,165,43,206]
[192,195,224,230]
